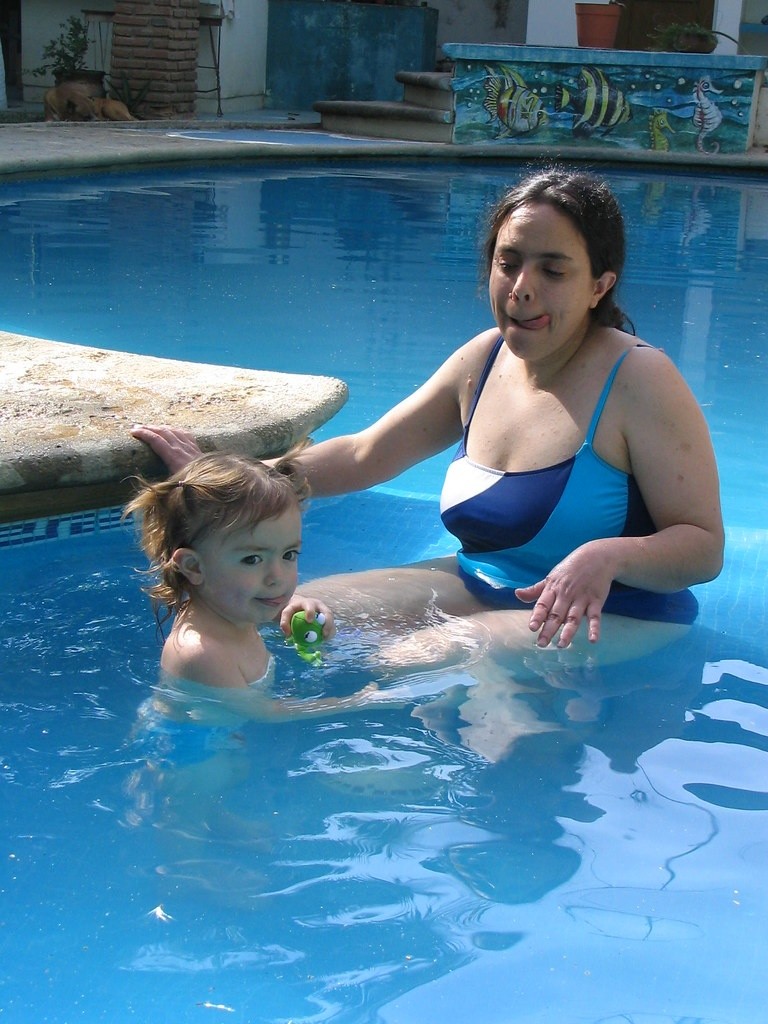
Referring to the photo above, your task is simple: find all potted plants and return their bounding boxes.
[649,22,717,53]
[23,15,106,119]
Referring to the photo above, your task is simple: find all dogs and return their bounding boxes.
[44,81,138,121]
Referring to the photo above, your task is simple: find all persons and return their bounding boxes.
[130,166,730,673]
[124,449,418,722]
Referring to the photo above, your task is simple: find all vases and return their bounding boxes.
[576,4,623,47]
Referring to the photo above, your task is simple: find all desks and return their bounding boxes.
[80,9,224,117]
